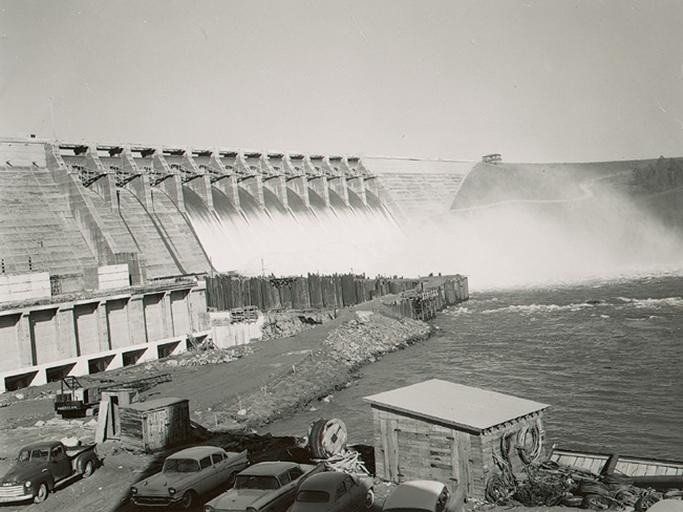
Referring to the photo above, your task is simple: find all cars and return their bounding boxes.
[379,476,469,512]
[203,460,316,512]
[129,445,251,509]
[286,471,377,512]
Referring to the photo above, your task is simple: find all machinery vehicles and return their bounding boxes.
[54,371,174,418]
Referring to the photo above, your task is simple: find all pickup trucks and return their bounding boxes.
[0,440,100,506]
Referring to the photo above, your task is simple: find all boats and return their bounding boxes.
[542,443,682,486]
